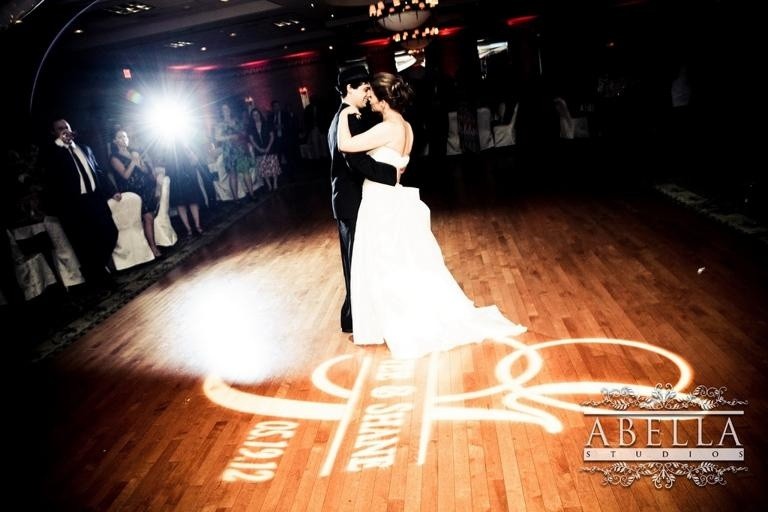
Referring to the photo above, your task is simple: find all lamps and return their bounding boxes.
[368,0,438,54]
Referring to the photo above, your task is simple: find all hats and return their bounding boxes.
[338,66,370,88]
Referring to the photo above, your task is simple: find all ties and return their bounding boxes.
[68,144,93,194]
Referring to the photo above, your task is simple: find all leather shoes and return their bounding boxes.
[186,228,206,239]
[153,252,166,259]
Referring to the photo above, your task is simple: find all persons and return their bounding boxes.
[0,57,642,363]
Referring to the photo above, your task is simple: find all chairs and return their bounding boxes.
[6,153,265,306]
[446,99,589,155]
